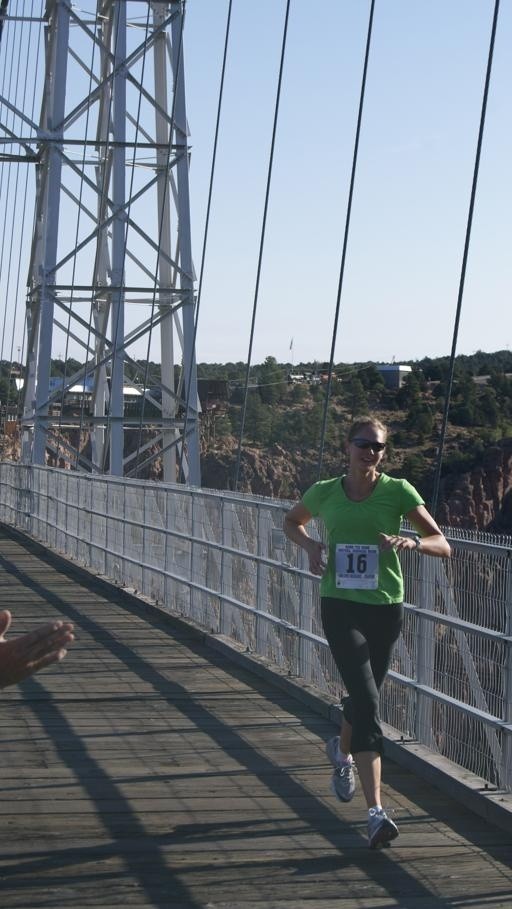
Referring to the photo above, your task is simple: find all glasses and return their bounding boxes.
[354,439,385,452]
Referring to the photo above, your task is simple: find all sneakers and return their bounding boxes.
[327,736,356,802]
[368,808,399,849]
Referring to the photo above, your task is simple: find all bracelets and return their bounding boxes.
[409,535,421,552]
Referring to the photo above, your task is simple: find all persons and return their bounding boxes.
[283,418,454,850]
[0,607,78,691]
